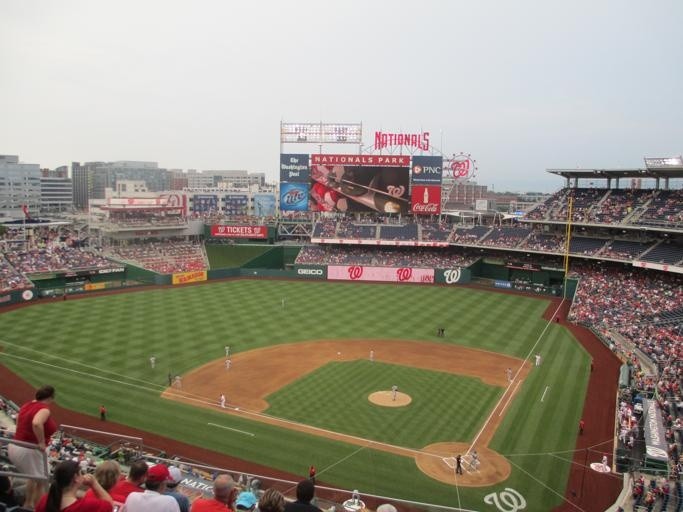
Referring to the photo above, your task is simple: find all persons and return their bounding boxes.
[453,454,462,474]
[502,365,511,383]
[166,370,173,386]
[174,374,181,388]
[468,449,478,471]
[224,344,230,358]
[62,292,66,301]
[367,345,373,361]
[225,357,231,372]
[149,354,154,370]
[390,382,397,401]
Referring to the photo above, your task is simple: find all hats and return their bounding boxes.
[145,464,183,484]
[236,492,257,509]
